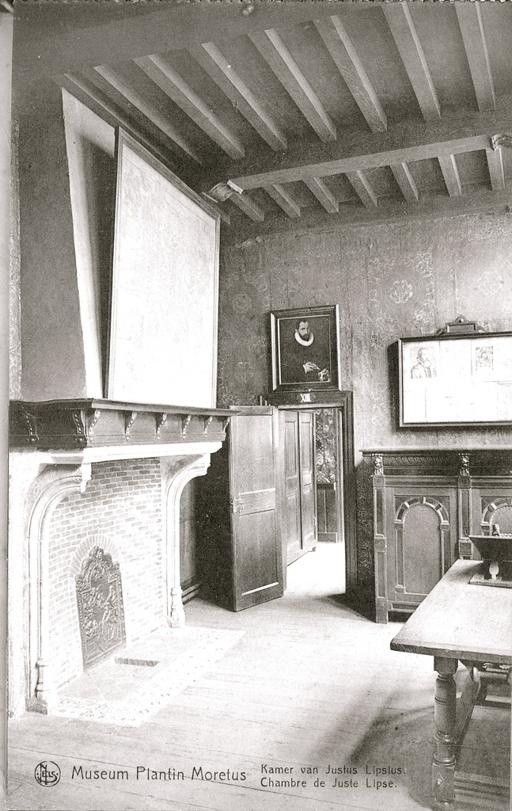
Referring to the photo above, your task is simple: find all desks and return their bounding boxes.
[389,560,511,810]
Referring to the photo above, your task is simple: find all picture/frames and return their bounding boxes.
[397,316,512,427]
[267,303,341,392]
[102,127,222,411]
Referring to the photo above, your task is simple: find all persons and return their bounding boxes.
[477,352,492,370]
[411,347,437,380]
[283,318,328,383]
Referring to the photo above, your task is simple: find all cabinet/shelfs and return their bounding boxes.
[282,410,317,564]
